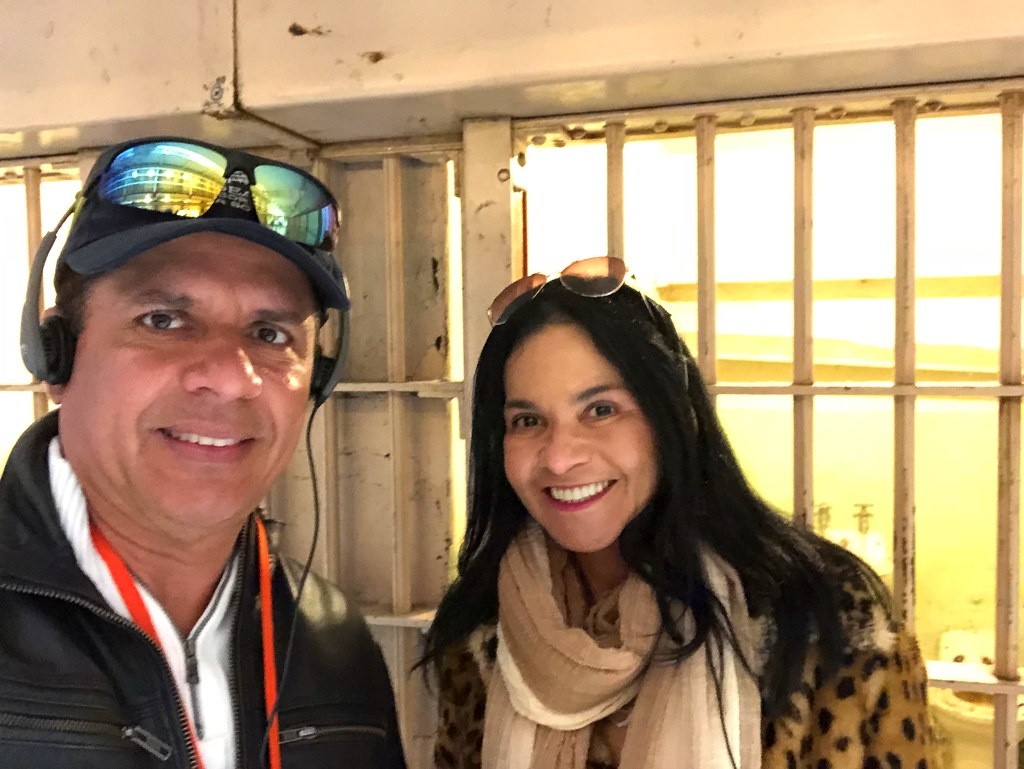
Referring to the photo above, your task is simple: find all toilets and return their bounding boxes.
[928,628,1024,769]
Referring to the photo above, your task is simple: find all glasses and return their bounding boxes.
[69,137,341,247]
[484,259,656,328]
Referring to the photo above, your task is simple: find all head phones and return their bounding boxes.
[20,202,351,405]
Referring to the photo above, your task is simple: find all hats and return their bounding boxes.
[53,137,347,309]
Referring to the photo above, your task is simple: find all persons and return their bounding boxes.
[1,134,410,769]
[421,257,946,769]
